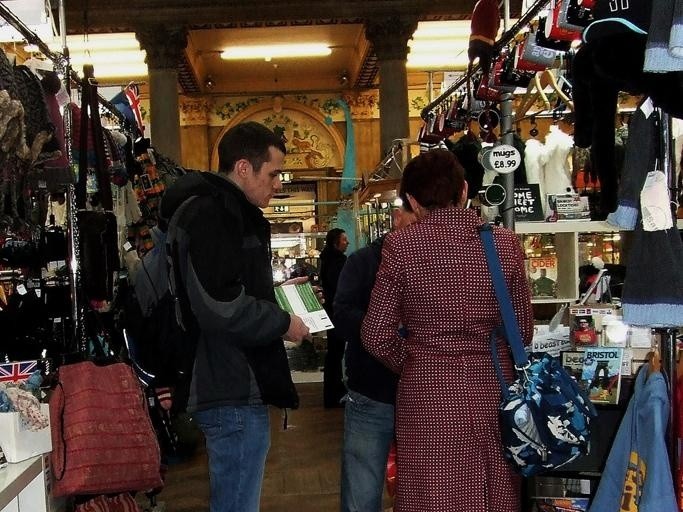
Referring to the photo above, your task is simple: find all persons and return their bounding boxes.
[320,228,349,407]
[334,190,418,512]
[360,148,534,512]
[169,120,325,512]
[571,319,596,345]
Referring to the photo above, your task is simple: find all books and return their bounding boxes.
[579,348,625,405]
[570,308,599,350]
[529,257,557,298]
[274,282,336,336]
[564,353,583,378]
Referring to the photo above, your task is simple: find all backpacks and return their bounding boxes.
[122,194,206,358]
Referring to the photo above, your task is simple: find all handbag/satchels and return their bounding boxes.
[478,222,598,474]
[51,308,163,495]
[87,267,114,314]
[77,64,121,271]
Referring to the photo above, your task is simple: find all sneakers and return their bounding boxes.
[325,392,347,407]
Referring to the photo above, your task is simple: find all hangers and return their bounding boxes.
[511,71,551,124]
[520,67,574,118]
[676,350,683,379]
[0,11,121,127]
[647,328,662,372]
